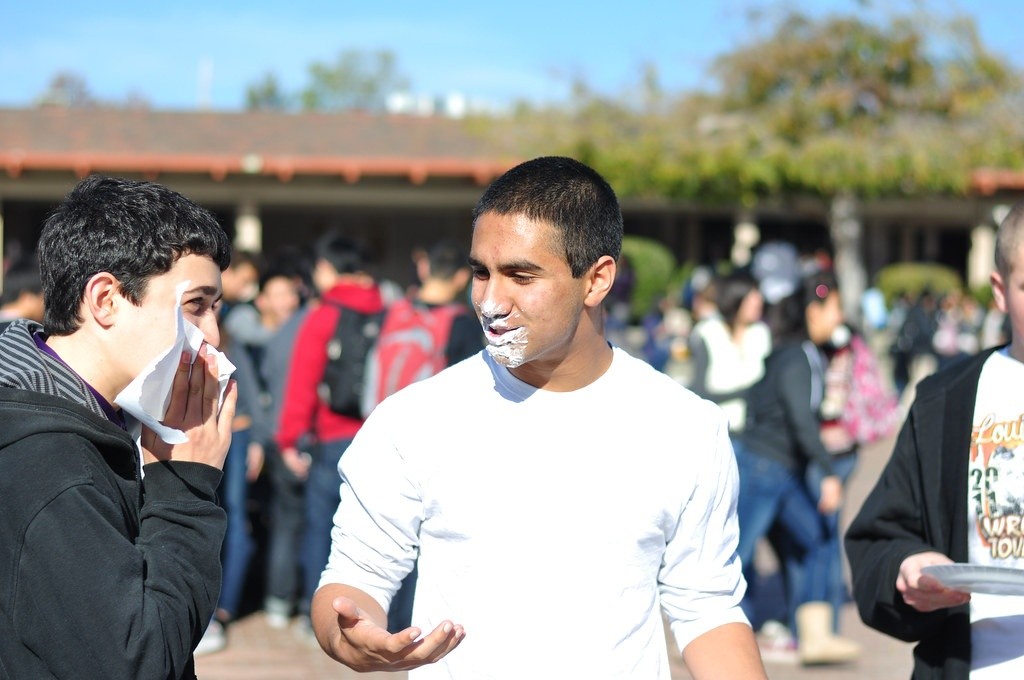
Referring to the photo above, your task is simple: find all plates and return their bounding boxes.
[919,563,1024,596]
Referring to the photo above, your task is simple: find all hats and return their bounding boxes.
[752,245,798,301]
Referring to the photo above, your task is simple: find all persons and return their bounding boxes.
[0,234,1009,669]
[0,177,238,680]
[310,157,768,680]
[845,201,1024,680]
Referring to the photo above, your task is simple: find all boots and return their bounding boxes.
[796,600,860,665]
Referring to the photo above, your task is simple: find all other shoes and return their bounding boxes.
[193,619,227,658]
[754,620,799,663]
[263,600,290,629]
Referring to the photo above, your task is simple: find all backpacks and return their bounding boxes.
[315,299,387,413]
[361,327,441,417]
